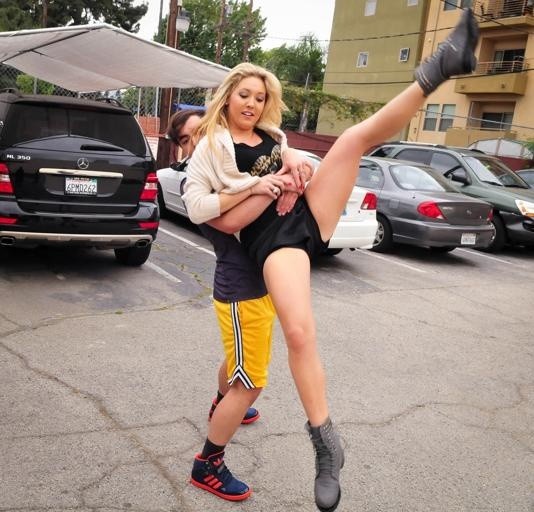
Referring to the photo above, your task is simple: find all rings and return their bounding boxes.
[273,187,280,193]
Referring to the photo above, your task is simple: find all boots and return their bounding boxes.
[301,418,344,507]
[410,7,481,97]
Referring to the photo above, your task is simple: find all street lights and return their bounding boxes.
[156,0,193,170]
[213,1,234,103]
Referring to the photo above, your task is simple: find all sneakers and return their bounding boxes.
[188,448,252,502]
[206,389,260,426]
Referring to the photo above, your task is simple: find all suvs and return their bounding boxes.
[367,141,534,254]
[0,85,161,265]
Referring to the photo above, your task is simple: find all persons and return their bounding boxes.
[163,107,315,503]
[183,4,481,511]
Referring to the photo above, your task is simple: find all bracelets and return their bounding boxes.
[250,186,254,194]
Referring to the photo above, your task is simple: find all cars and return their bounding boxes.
[356,155,495,251]
[467,138,534,187]
[156,145,378,256]
